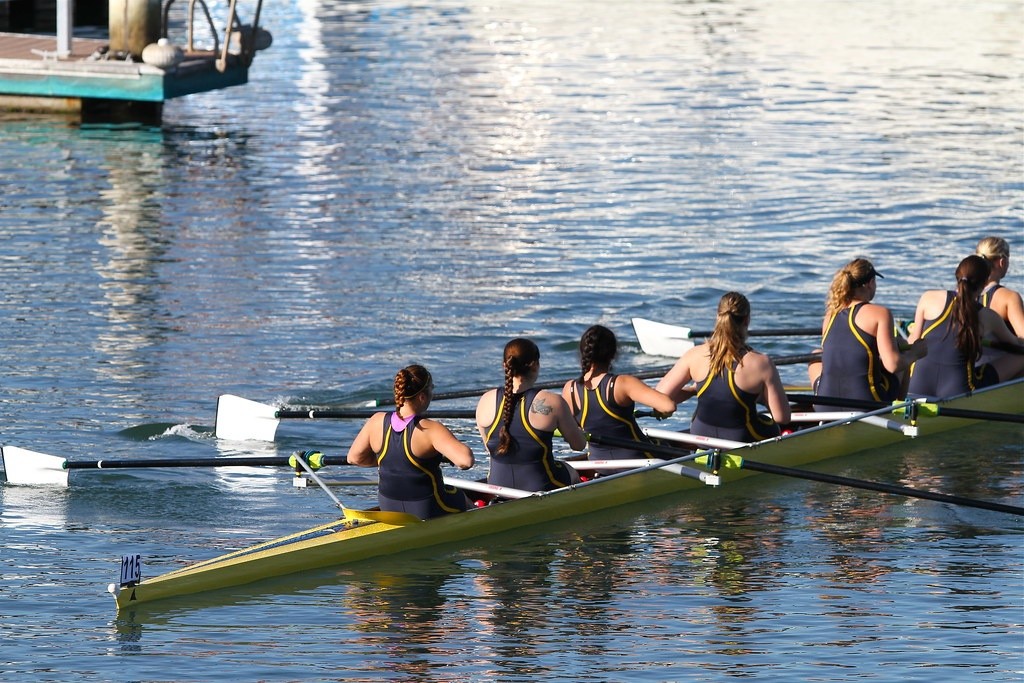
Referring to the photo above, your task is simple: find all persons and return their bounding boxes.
[346,365,477,523]
[655,292,791,442]
[955,236,1024,340]
[907,255,1022,401]
[475,338,587,491]
[562,325,677,476]
[812,258,927,416]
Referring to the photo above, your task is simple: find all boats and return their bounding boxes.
[108,368,1024,614]
[0,0,275,132]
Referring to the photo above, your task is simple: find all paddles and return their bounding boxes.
[692,446,1024,516]
[890,400,1024,424]
[2,441,350,487]
[369,351,824,410]
[629,316,908,357]
[213,392,659,443]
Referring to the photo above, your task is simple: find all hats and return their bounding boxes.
[850,266,884,288]
